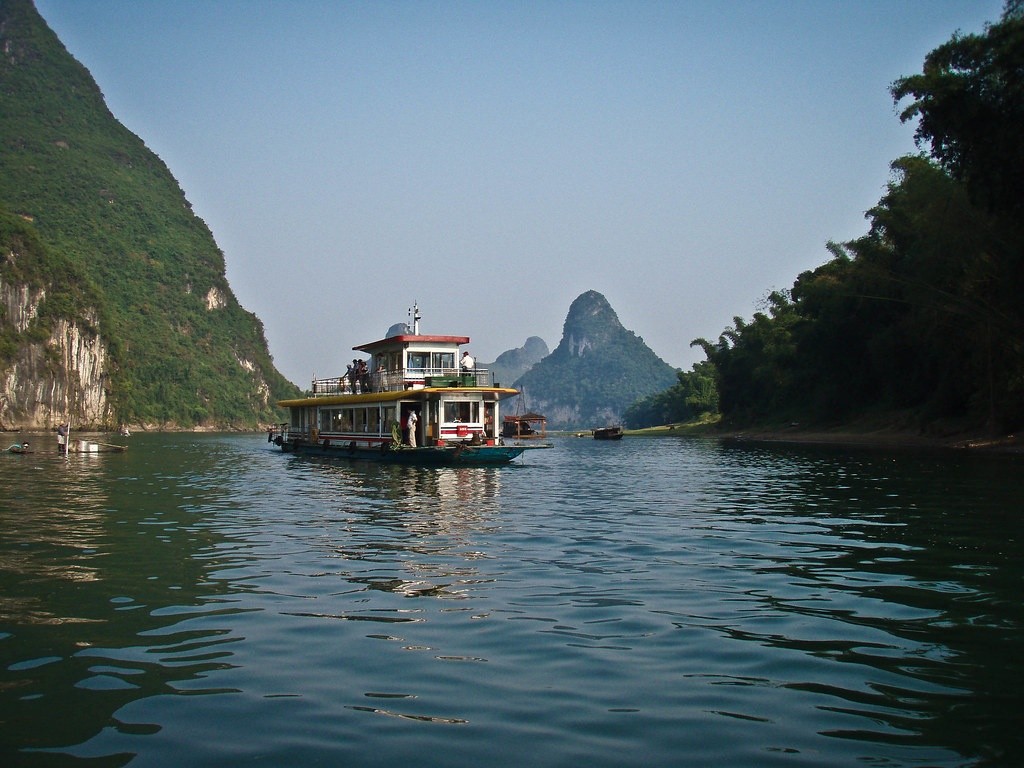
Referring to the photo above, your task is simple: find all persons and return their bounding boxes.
[407,407,418,448]
[343,364,357,395]
[58,421,68,452]
[352,359,372,394]
[376,366,388,392]
[460,351,474,376]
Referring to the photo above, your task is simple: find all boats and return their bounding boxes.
[268,299,554,466]
[502,385,547,439]
[8,445,127,453]
[594,425,624,440]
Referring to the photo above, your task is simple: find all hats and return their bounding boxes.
[463,351,469,354]
